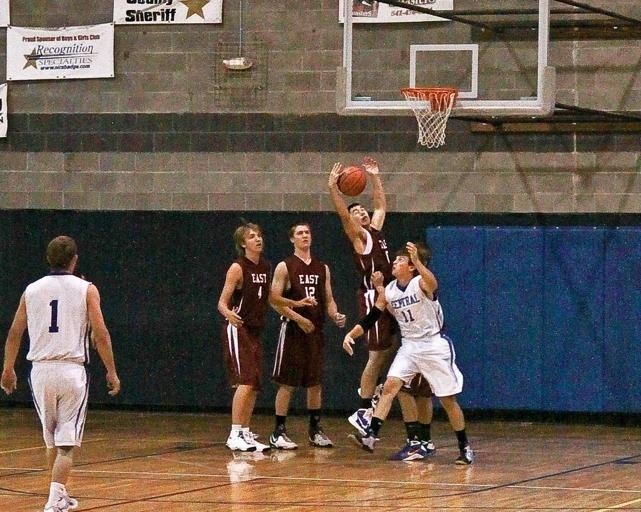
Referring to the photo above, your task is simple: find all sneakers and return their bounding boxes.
[43,487,79,512]
[242,430,271,453]
[346,430,380,454]
[308,431,334,449]
[224,425,257,452]
[268,431,299,451]
[350,408,377,437]
[389,435,427,462]
[397,439,436,457]
[453,446,475,466]
[356,383,384,409]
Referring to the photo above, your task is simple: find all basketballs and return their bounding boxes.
[338,166,367,196]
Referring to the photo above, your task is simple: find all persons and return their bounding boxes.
[370,241,434,461]
[328,155,399,441]
[0,235,121,512]
[269,221,346,450]
[343,241,475,466]
[217,223,315,452]
